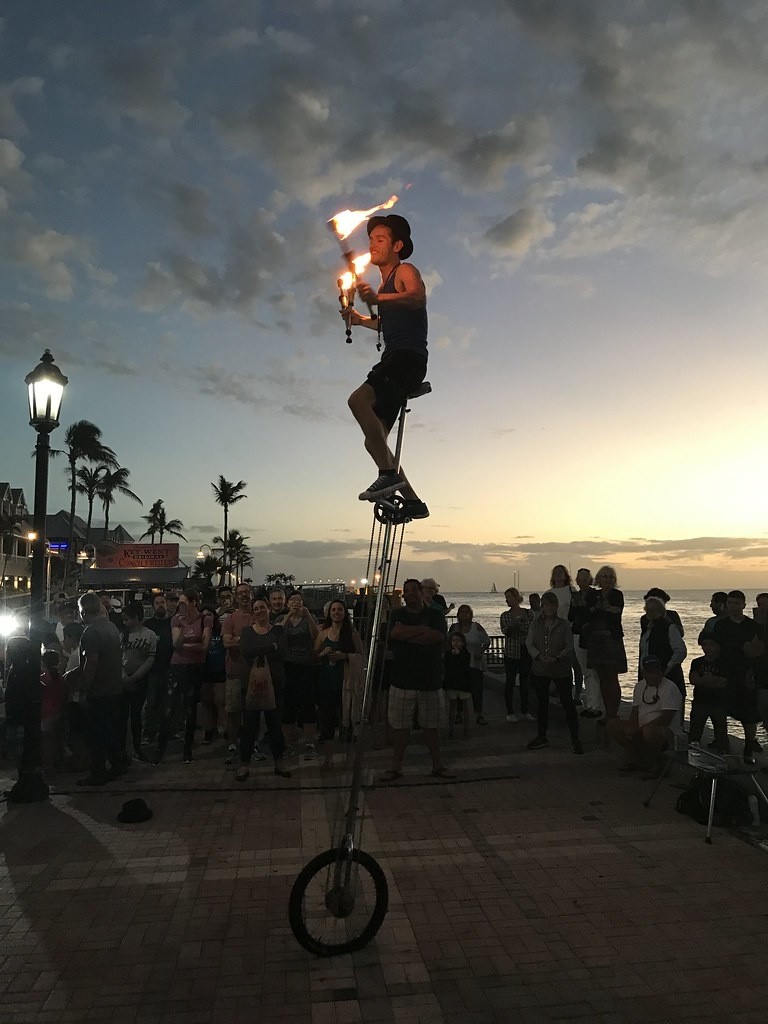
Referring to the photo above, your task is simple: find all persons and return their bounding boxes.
[526,592,585,755]
[236,597,291,780]
[637,588,768,765]
[5,583,454,766]
[339,213,429,519]
[448,605,491,726]
[62,593,124,786]
[442,632,476,739]
[605,655,683,779]
[501,566,627,726]
[381,579,457,782]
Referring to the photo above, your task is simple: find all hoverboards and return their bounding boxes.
[288,382,432,957]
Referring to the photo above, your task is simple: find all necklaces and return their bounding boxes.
[376,263,401,351]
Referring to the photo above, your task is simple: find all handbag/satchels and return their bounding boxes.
[241,657,277,712]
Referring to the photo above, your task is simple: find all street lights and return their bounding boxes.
[6,347,69,806]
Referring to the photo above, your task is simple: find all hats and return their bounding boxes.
[117,798,153,824]
[642,655,661,673]
[367,215,413,260]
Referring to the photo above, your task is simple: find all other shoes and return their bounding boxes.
[526,734,550,750]
[743,739,764,752]
[707,743,730,754]
[379,769,401,782]
[571,741,584,754]
[506,714,518,722]
[431,767,453,783]
[688,741,700,749]
[476,716,488,726]
[521,714,536,722]
[358,472,406,500]
[744,742,757,766]
[384,503,430,519]
[411,723,424,734]
[570,698,622,726]
[69,728,266,787]
[273,760,292,778]
[455,715,463,724]
